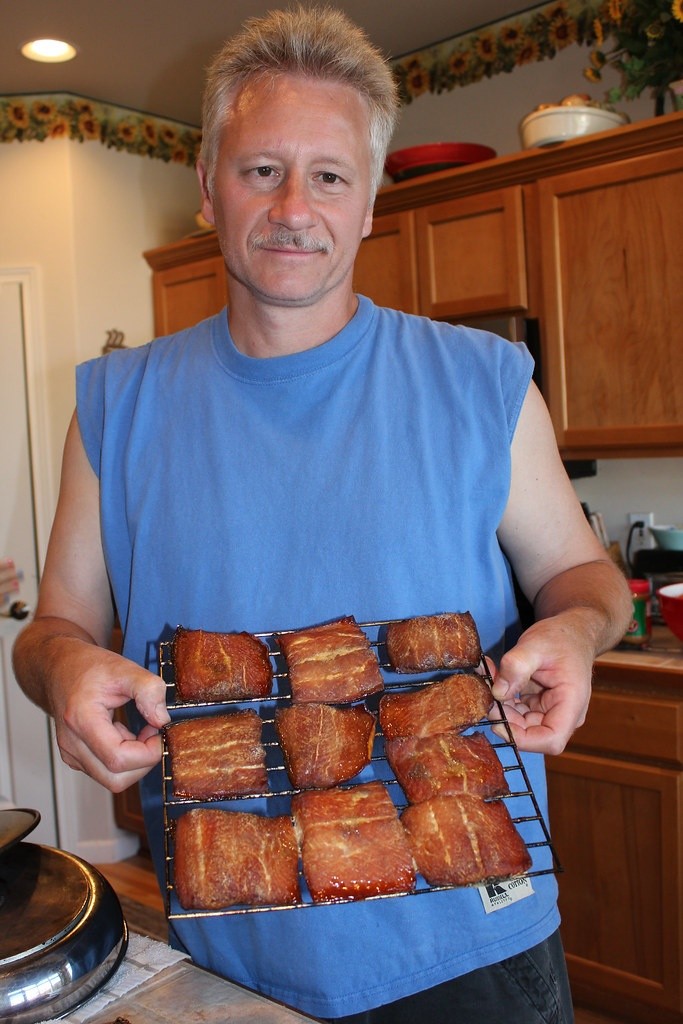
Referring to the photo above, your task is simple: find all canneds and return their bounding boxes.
[622,579,652,644]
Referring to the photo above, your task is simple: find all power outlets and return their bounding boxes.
[629,513,654,552]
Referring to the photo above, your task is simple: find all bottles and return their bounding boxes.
[620,579,653,650]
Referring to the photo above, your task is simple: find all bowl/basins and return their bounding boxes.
[384,142,497,182]
[647,525,683,551]
[518,105,629,150]
[655,581,683,647]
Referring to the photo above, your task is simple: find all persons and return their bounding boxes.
[10,6,630,1024]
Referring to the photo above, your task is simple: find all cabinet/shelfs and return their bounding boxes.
[537,660,683,1024]
[141,108,683,461]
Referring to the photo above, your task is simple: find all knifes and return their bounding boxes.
[579,500,610,549]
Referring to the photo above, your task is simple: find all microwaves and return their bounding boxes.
[456,316,598,480]
